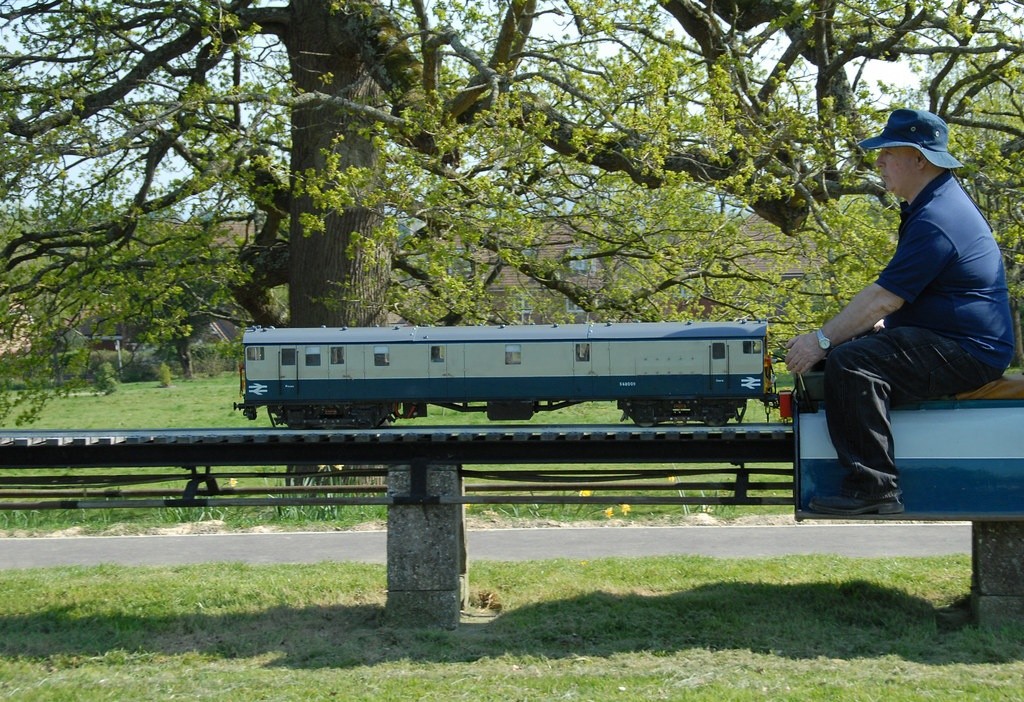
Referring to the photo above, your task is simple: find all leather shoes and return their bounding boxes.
[809,493,904,515]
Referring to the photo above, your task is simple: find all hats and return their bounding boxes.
[858,108,964,168]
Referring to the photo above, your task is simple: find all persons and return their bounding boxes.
[785,110,1014,515]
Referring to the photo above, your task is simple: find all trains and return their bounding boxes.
[233,318,772,429]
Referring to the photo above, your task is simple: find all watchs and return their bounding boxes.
[816,329,833,352]
